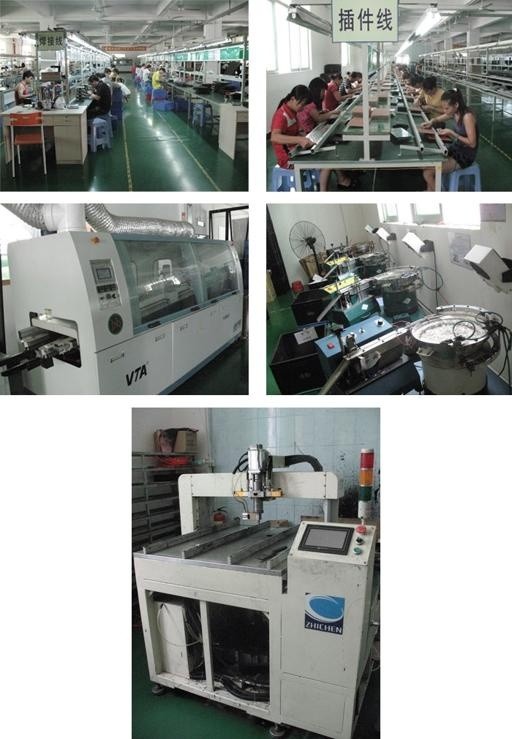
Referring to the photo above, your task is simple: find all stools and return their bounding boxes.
[98,115,114,138]
[442,161,481,191]
[193,103,213,128]
[272,164,314,192]
[88,117,112,152]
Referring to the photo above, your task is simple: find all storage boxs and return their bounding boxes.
[154,428,197,452]
[292,290,327,325]
[269,324,327,395]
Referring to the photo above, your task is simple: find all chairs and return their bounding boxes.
[9,112,48,179]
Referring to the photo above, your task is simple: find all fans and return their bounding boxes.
[289,220,326,275]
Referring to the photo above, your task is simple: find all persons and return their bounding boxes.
[391,60,478,191]
[271,68,362,190]
[87,63,170,134]
[1,63,39,105]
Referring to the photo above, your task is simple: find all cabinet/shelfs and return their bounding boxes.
[132,452,201,606]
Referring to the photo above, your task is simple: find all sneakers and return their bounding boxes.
[337,180,358,190]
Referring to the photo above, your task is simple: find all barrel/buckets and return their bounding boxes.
[410,316,491,395]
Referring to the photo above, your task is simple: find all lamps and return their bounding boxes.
[365,224,512,294]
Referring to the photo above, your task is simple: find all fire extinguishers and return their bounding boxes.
[213,505,228,526]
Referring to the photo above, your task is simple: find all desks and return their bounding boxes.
[167,80,238,103]
[288,87,449,192]
[71,97,93,106]
[0,106,88,165]
[218,103,248,160]
